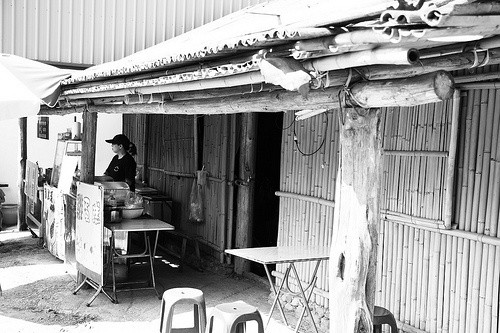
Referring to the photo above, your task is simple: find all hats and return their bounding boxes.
[105,133,130,146]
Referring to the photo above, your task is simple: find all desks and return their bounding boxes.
[225,244,331,333]
[72,182,174,307]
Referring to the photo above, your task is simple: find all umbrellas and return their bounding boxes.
[0,53,72,122]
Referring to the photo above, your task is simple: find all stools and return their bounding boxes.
[209,300,264,333]
[373,305,398,333]
[159,288,207,333]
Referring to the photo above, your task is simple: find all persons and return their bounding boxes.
[98,134,138,263]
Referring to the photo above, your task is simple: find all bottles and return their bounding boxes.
[111,206,118,222]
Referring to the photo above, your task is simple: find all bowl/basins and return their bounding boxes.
[119,206,143,220]
